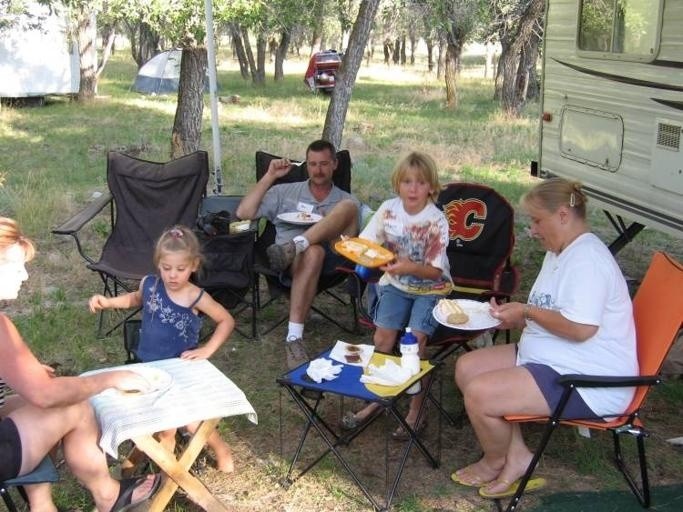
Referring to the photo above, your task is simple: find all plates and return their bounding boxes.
[116,364,173,399]
[274,212,325,226]
[431,297,504,331]
[334,234,394,269]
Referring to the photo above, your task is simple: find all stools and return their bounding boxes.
[271,346,447,512]
[78,357,259,512]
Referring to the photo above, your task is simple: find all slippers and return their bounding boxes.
[96,473,160,512]
[340,409,373,431]
[392,419,427,439]
[450,464,547,498]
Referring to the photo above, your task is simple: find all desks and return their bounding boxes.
[190,194,257,340]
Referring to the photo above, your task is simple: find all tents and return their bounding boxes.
[303,48,343,92]
[131,49,222,96]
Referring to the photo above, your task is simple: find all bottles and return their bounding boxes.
[399,324,423,397]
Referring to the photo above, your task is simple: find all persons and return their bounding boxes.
[340,151,455,443]
[236,140,361,372]
[1,217,160,512]
[451,177,641,499]
[89,224,233,472]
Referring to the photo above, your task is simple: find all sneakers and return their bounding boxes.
[285,338,310,372]
[266,238,296,270]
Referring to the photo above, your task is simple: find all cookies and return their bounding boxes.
[447,313,469,325]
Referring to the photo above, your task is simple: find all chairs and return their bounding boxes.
[493,249,683,512]
[249,148,366,336]
[0,453,61,512]
[120,319,187,474]
[335,181,521,417]
[48,149,210,334]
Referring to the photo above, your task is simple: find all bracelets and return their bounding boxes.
[523,303,530,322]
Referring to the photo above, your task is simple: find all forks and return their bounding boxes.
[291,159,306,167]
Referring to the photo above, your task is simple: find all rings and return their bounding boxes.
[494,312,500,320]
